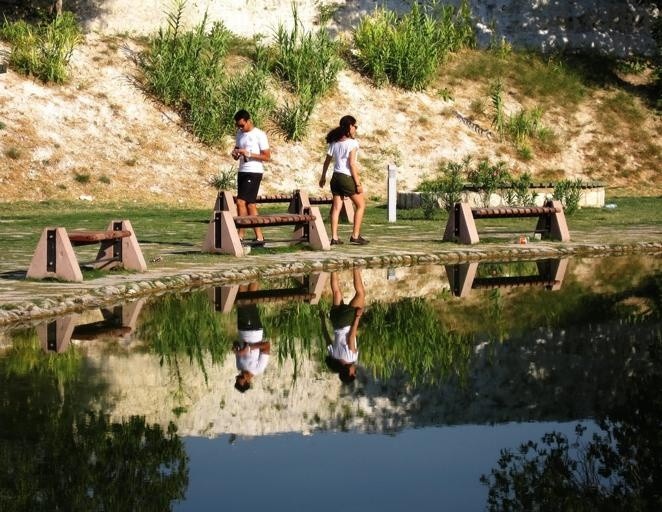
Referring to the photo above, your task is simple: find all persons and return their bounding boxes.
[232,282,270,392]
[232,110,270,246]
[319,115,370,245]
[321,267,366,383]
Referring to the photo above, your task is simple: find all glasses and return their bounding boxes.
[237,124,244,128]
[353,125,357,129]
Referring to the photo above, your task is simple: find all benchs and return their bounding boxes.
[201,191,356,258]
[25,219,145,281]
[208,272,327,315]
[442,200,573,244]
[36,296,150,354]
[444,258,570,296]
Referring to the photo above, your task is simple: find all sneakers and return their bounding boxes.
[331,239,343,245]
[350,235,369,244]
[251,239,265,247]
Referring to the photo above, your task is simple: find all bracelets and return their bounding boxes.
[248,344,251,352]
[248,151,252,158]
[357,183,362,187]
[356,315,361,317]
